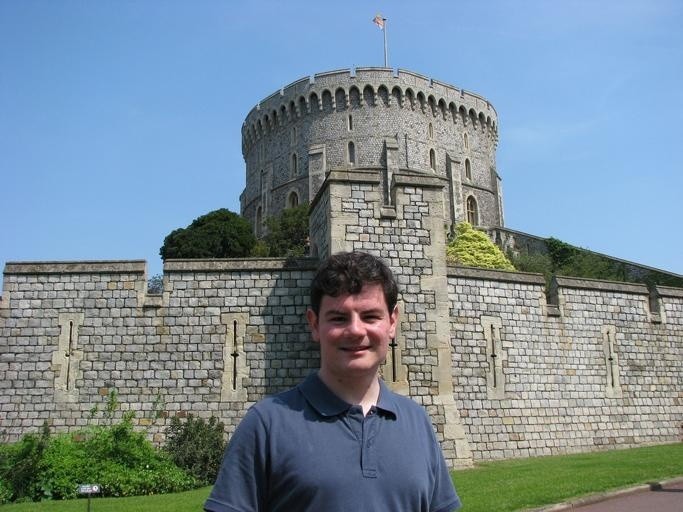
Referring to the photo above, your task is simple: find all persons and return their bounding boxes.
[201,249,464,512]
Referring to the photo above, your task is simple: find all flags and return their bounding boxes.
[372,15,385,29]
